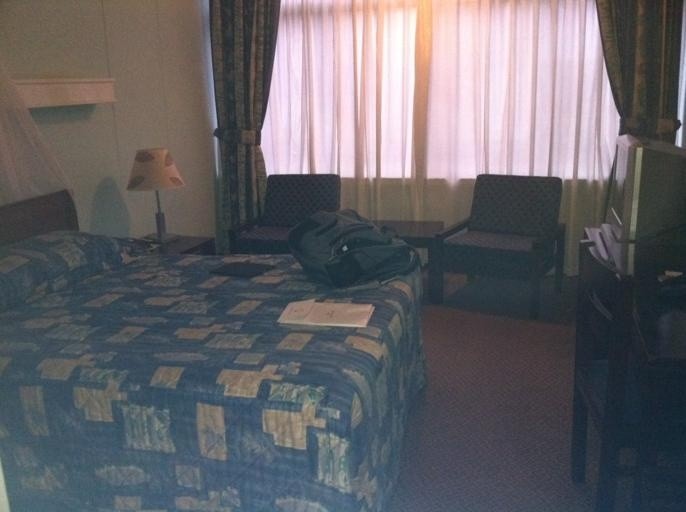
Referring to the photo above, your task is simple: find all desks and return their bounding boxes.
[585,222,685,511]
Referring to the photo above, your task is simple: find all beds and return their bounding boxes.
[1,187,430,512]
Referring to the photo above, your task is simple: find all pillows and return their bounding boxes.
[0,232,143,314]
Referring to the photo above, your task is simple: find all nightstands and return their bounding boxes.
[138,230,216,259]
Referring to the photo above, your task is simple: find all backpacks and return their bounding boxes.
[285,209,418,293]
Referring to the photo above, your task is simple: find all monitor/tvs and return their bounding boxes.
[600,134,686,283]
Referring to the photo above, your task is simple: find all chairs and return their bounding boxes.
[564,239,634,509]
[433,173,569,319]
[228,174,340,257]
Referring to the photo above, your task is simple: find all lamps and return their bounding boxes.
[124,144,185,246]
